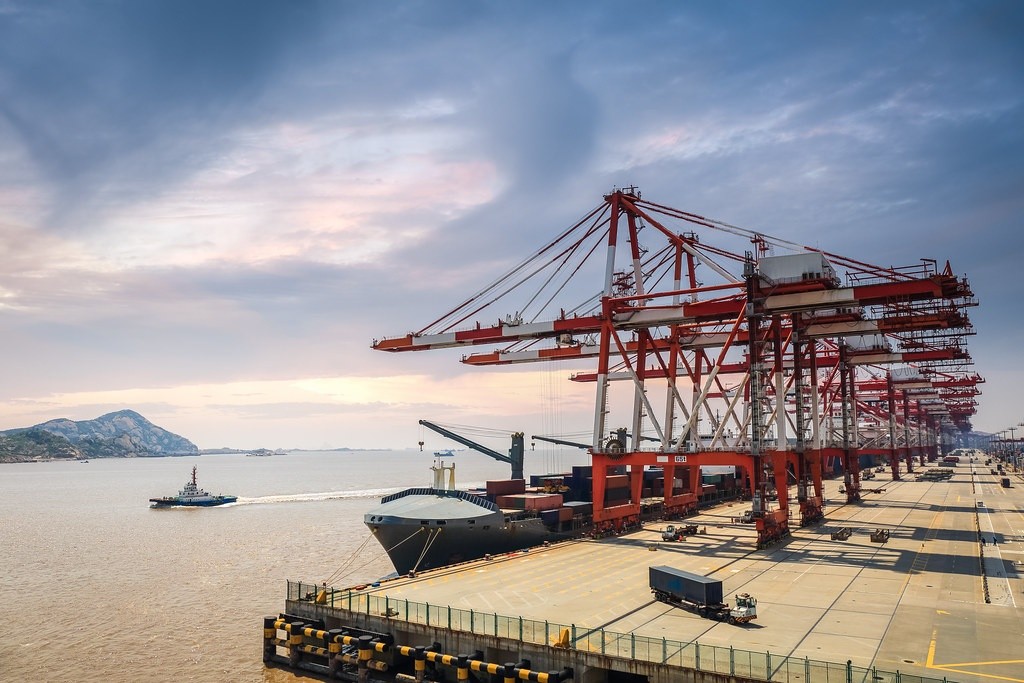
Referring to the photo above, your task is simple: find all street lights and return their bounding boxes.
[1008,427,1018,455]
[989,430,1009,456]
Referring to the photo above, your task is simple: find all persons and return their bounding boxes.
[876,529,879,533]
[162,495,165,500]
[981,536,987,546]
[166,497,168,500]
[991,536,998,547]
[881,530,886,535]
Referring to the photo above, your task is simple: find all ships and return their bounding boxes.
[362,418,740,577]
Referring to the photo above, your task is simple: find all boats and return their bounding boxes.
[245,453,287,457]
[433,451,454,456]
[149,467,238,507]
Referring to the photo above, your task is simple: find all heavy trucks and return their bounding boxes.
[662,525,697,541]
[985,459,1010,489]
[742,510,765,523]
[648,564,758,626]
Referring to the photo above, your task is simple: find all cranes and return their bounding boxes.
[370,184,986,549]
